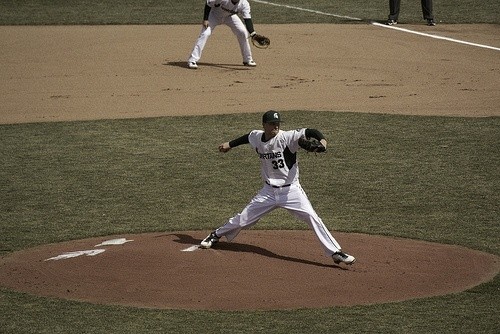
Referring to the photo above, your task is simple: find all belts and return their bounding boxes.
[266,181,291,188]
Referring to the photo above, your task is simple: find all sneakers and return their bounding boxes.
[244,60,256,66]
[385,19,397,25]
[187,62,197,69]
[200,233,217,248]
[427,19,436,26]
[332,251,356,265]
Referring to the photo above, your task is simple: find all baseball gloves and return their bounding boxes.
[251,33,271,50]
[298,136,327,153]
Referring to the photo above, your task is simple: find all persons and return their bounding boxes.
[387,0,436,26]
[201,110,356,265]
[187,0,270,68]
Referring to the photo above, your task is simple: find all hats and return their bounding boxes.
[262,110,284,123]
